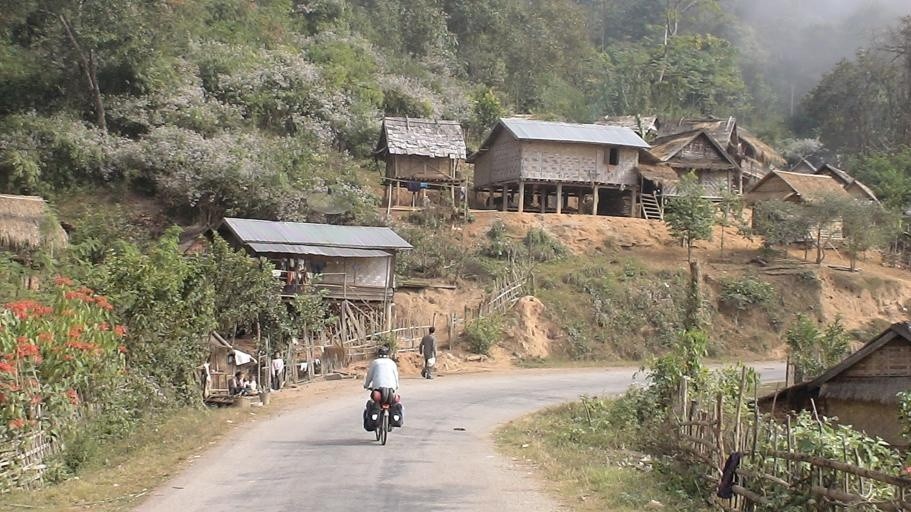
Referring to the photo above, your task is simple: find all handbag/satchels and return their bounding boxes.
[364,401,382,431]
[390,403,404,427]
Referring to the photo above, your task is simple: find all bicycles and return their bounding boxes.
[363,386,400,445]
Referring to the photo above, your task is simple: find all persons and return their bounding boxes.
[228,371,244,394]
[238,375,257,396]
[364,346,399,392]
[271,351,284,390]
[420,327,436,379]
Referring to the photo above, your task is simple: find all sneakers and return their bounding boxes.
[422,370,426,377]
[427,374,433,379]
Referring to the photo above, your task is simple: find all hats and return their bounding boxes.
[379,346,387,355]
